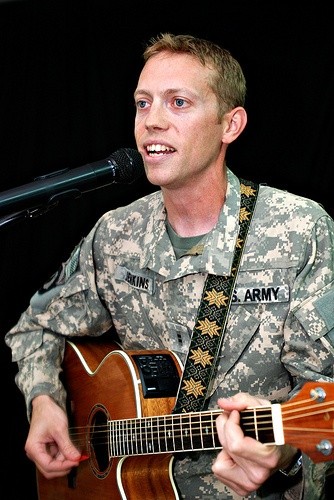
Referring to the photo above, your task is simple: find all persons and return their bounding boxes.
[4,33,334,500]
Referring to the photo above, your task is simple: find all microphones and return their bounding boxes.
[0,149,144,219]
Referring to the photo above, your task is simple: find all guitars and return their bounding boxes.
[36,339,333,499]
